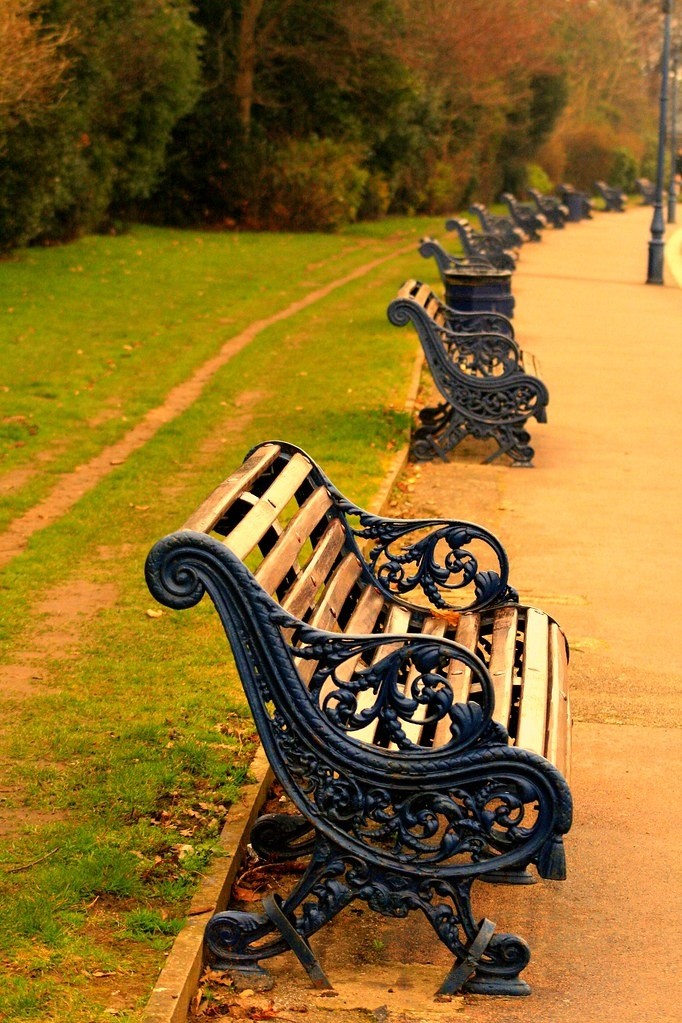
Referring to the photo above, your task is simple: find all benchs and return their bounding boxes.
[386,278,550,468]
[146,438,573,997]
[417,176,669,319]
